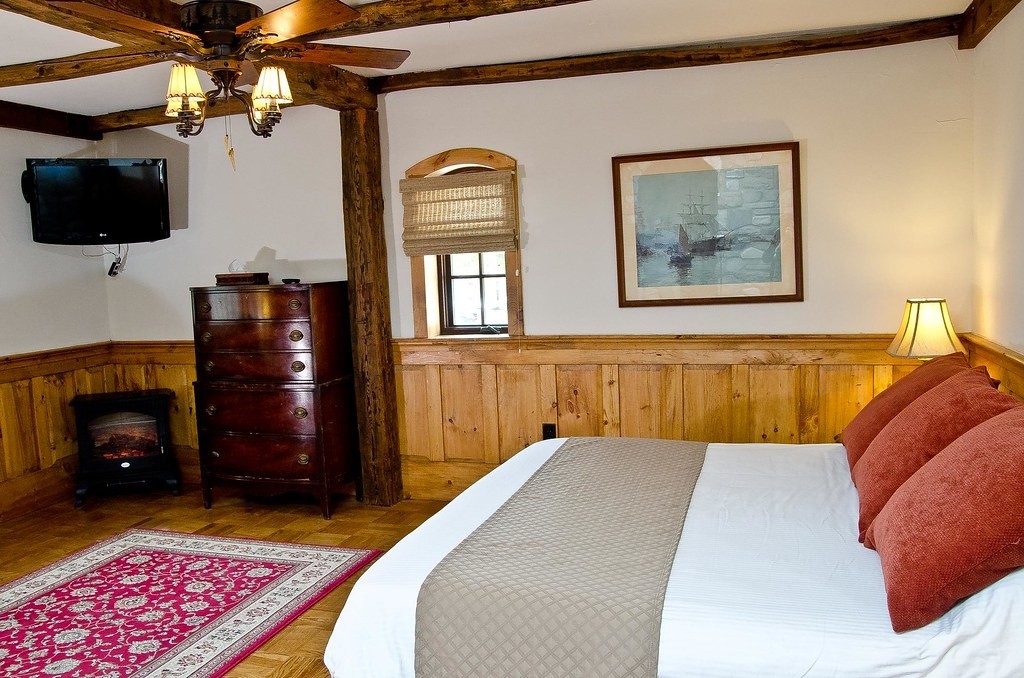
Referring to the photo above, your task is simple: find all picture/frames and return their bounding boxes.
[612,141,804,308]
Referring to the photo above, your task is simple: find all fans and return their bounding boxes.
[0,0,411,87]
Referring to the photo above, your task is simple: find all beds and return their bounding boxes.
[322,436,1024,678]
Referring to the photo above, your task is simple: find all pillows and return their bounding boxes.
[832,351,976,470]
[863,404,1024,633]
[852,365,1022,543]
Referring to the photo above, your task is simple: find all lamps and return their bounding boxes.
[163,57,294,138]
[883,298,971,365]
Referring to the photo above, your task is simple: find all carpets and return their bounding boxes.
[0,528,385,678]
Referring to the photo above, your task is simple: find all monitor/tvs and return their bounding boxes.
[26,157,171,245]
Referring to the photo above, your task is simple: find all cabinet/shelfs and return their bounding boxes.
[189,280,364,521]
